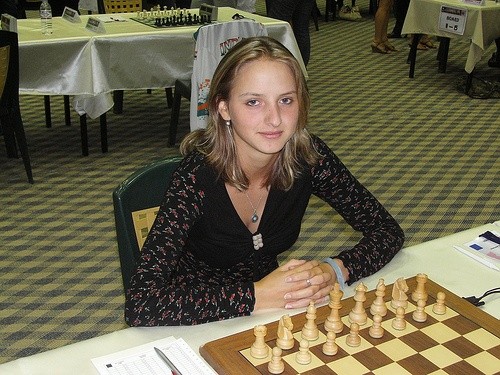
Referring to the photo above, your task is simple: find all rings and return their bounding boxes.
[306,280,311,286]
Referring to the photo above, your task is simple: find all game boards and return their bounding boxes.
[198,275,499,375]
[129,13,212,29]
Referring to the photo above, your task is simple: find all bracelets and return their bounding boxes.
[323,257,344,291]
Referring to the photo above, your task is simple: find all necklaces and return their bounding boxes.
[244,188,264,224]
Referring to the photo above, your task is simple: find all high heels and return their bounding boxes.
[383,40,398,52]
[371,40,389,54]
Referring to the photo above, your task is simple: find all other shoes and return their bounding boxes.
[368,5,378,20]
[487,52,497,67]
[335,6,357,21]
[386,32,402,38]
[351,6,362,21]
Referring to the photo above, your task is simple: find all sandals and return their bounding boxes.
[409,41,428,51]
[421,40,437,50]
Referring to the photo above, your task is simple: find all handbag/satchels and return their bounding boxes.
[458,72,498,100]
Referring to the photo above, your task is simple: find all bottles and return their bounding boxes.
[40,0,53,35]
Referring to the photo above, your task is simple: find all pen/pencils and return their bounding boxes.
[110,17,119,21]
[152,346,182,375]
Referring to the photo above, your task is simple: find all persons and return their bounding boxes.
[410,34,432,49]
[125,36,405,328]
[387,0,410,38]
[266,0,313,68]
[370,0,396,54]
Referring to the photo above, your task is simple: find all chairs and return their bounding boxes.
[113,156,182,294]
[0,30,36,184]
[168,17,268,146]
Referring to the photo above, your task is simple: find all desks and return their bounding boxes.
[0,221,500,375]
[401,0,500,94]
[18,6,308,157]
